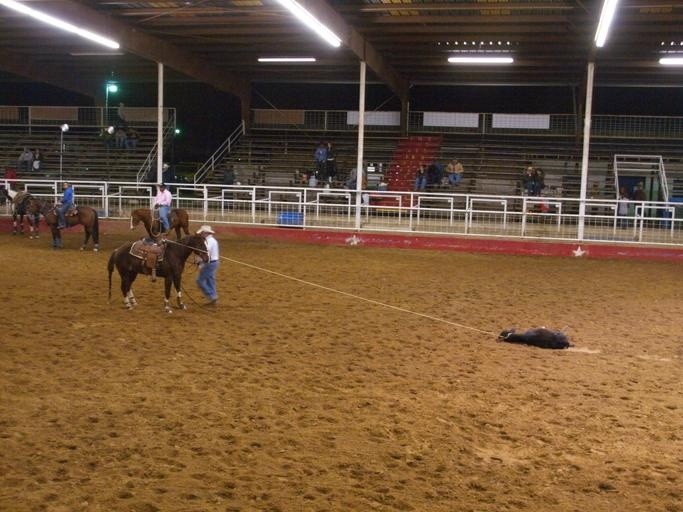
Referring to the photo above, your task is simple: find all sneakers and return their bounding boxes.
[204,296,217,304]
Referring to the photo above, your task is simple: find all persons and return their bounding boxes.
[14,146,33,172]
[445,156,464,187]
[532,168,544,198]
[411,164,428,193]
[631,184,646,223]
[115,129,125,148]
[123,127,139,149]
[615,184,630,201]
[153,183,172,233]
[195,224,221,306]
[313,141,327,178]
[53,182,73,230]
[31,148,43,174]
[617,194,630,231]
[293,166,367,204]
[631,179,646,200]
[521,166,536,196]
[426,160,444,188]
[325,142,337,178]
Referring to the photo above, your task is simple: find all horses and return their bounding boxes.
[107,232,210,315]
[25,198,99,252]
[0,186,40,240]
[130,208,190,241]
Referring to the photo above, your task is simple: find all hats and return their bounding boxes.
[196,225,214,234]
[157,182,165,187]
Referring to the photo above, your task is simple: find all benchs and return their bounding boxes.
[194,128,683,225]
[1,123,171,208]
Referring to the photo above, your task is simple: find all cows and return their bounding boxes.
[498,326,571,349]
